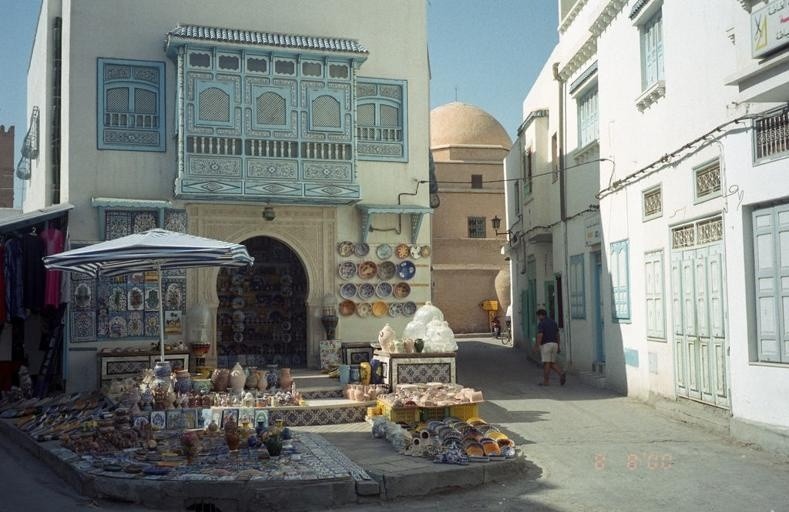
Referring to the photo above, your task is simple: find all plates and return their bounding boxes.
[102,464,143,473]
[427,417,515,458]
[231,274,294,344]
[338,240,431,317]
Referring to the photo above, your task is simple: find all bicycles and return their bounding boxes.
[502,320,511,345]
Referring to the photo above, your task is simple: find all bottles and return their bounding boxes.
[339,359,391,402]
[209,415,294,459]
[110,362,305,413]
[221,345,305,367]
[378,323,425,353]
[372,415,412,454]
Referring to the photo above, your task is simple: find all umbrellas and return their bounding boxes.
[41,226,256,364]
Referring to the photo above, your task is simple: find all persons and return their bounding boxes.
[534,310,567,386]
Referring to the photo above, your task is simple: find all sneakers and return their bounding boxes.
[560,370,567,386]
[538,382,550,386]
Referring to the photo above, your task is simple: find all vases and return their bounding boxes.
[321,315,424,401]
[110,361,292,466]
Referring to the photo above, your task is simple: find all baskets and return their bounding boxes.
[368,397,479,425]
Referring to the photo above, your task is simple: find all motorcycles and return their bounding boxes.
[491,316,500,338]
